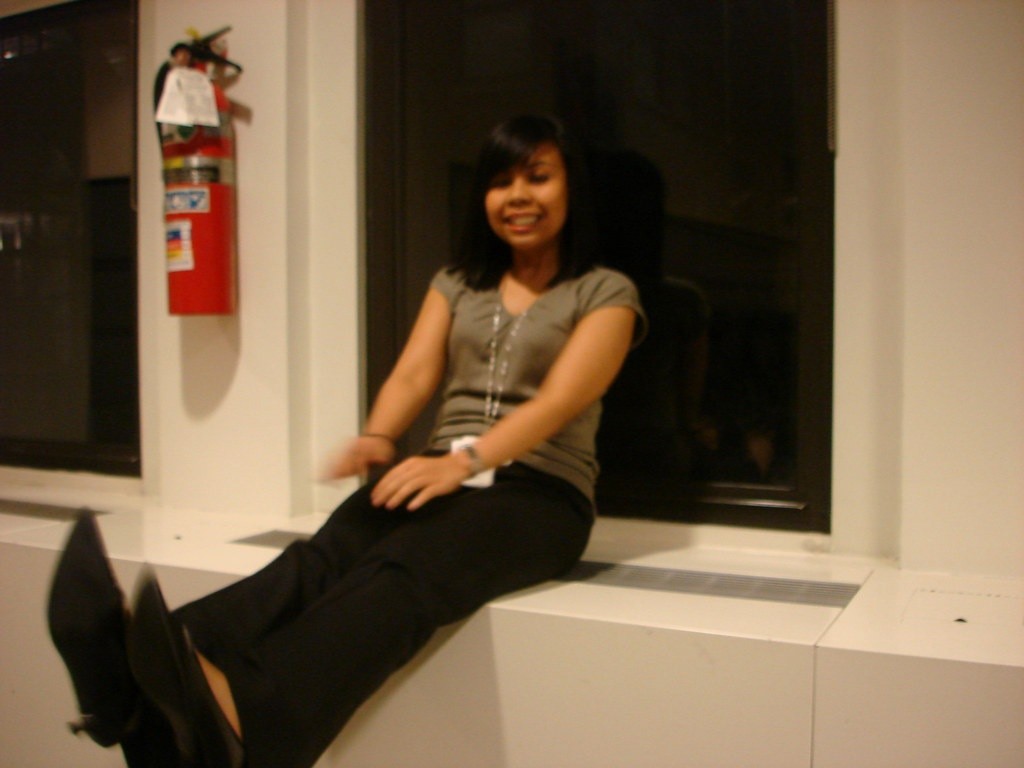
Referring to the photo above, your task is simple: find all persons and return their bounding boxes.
[45,105,638,768]
[571,148,773,517]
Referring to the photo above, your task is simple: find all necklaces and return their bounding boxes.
[484,295,529,421]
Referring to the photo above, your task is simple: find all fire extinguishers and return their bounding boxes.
[154,24,241,317]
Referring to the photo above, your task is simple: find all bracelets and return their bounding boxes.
[460,445,485,475]
[358,434,396,449]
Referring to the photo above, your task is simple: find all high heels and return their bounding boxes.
[44,507,135,748]
[124,576,248,768]
